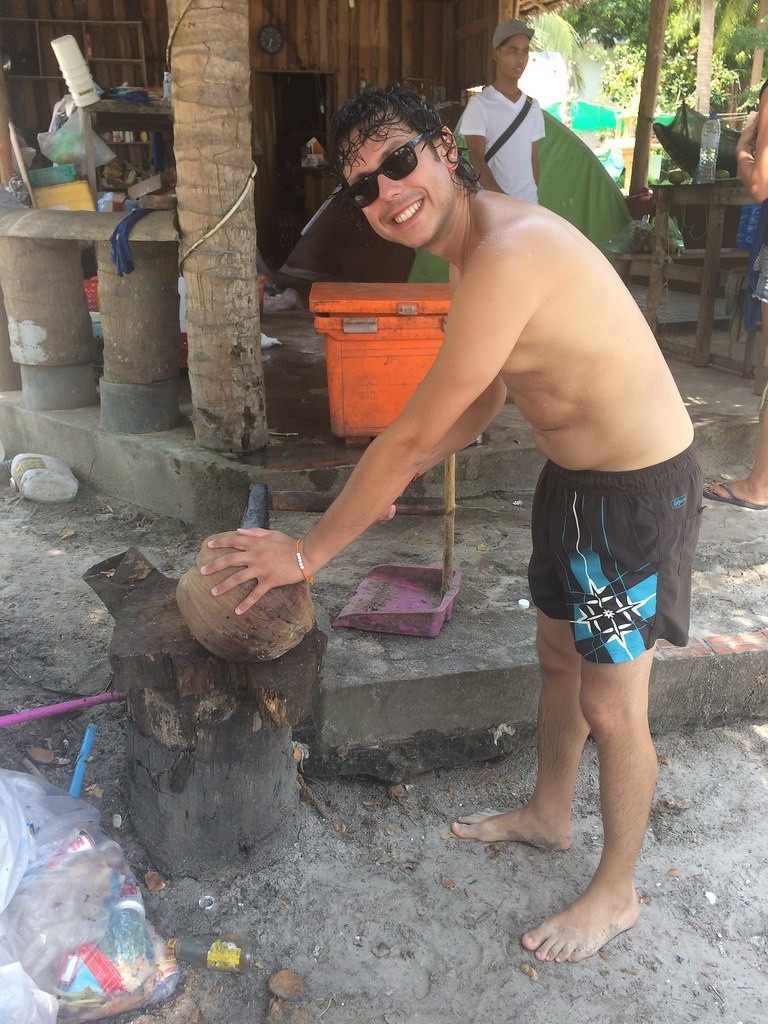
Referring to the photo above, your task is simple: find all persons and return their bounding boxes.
[458,18,545,204]
[201,85,702,961]
[702,79,768,510]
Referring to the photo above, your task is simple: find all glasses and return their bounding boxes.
[335,119,452,210]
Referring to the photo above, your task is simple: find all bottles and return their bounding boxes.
[9,452,79,503]
[696,111,721,185]
[163,935,254,974]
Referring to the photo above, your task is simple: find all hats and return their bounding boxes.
[491,18,535,48]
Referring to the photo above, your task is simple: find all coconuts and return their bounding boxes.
[178,531,314,662]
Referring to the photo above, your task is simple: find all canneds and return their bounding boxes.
[114,886,145,917]
[150,931,180,1000]
[99,842,123,865]
[51,831,93,863]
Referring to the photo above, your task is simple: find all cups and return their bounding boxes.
[50,33,101,109]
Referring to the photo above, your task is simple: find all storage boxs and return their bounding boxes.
[310,281,455,448]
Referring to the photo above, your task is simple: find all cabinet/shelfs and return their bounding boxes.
[0,17,174,211]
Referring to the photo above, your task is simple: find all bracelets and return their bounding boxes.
[296,539,313,586]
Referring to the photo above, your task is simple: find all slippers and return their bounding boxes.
[702,479,768,510]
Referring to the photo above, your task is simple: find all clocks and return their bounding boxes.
[258,25,285,54]
